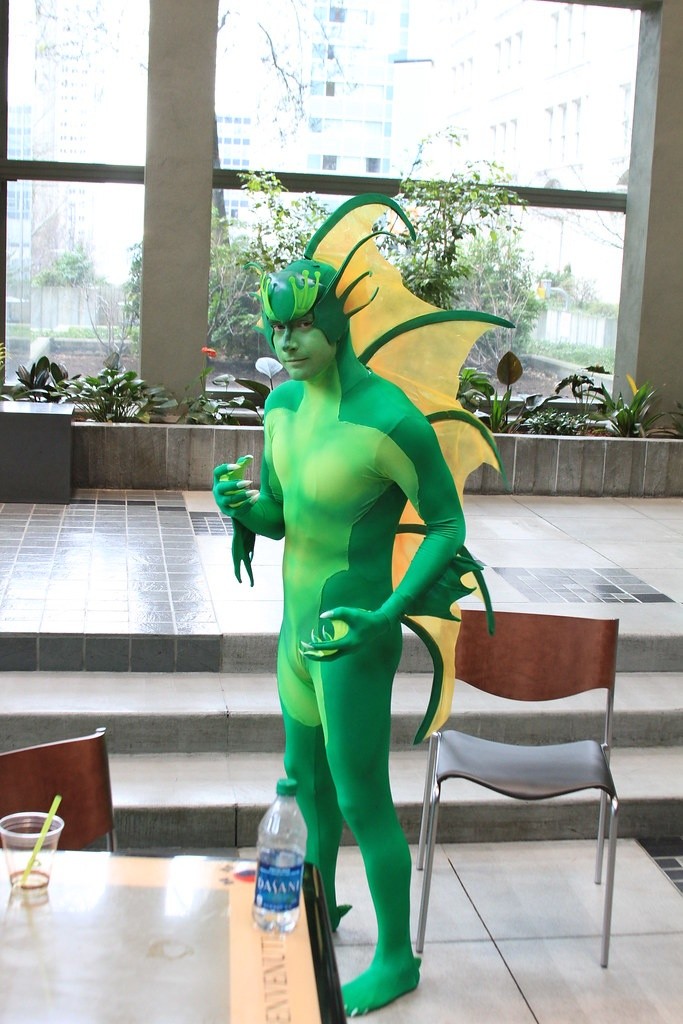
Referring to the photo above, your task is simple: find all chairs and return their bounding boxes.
[414,609,619,969]
[0,726,119,853]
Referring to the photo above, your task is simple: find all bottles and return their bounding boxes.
[252,778,309,931]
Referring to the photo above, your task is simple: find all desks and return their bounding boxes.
[1,847,347,1022]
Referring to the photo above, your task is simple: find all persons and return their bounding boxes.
[212,259,466,1016]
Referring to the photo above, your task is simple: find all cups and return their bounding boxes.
[0,812,65,895]
